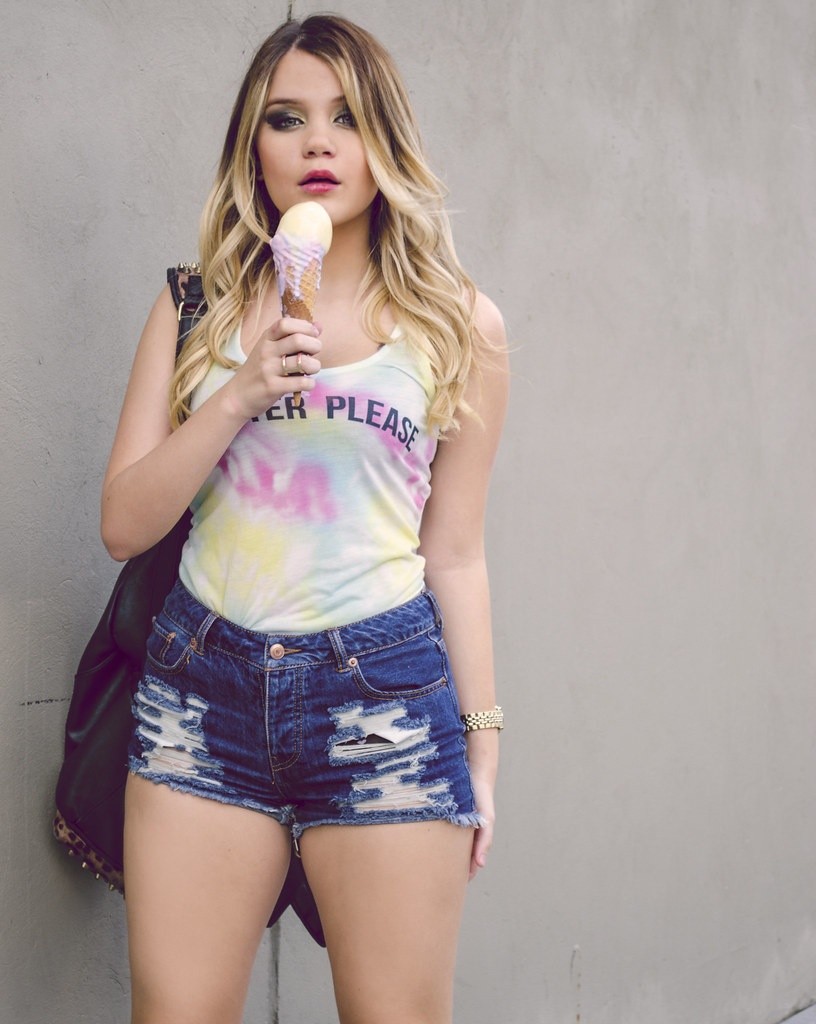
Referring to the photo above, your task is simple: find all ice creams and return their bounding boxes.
[270,200,333,407]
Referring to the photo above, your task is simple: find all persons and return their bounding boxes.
[97,12,508,1022]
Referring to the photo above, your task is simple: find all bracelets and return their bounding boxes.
[460,706,505,733]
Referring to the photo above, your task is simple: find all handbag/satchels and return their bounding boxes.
[53,262,326,947]
